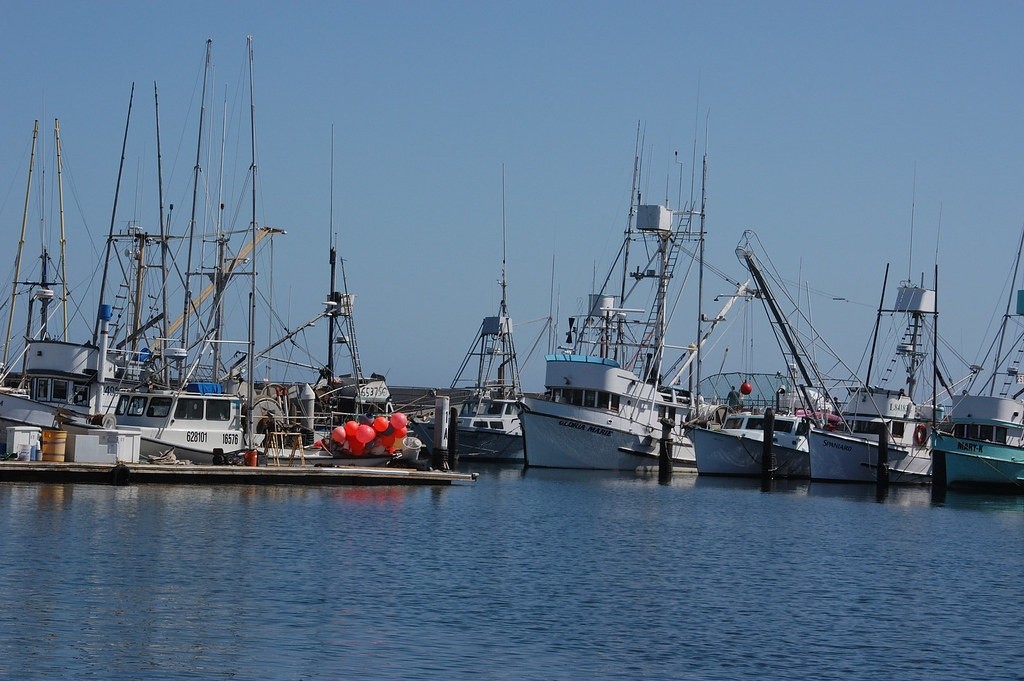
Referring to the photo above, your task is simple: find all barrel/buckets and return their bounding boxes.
[36,450,43,461]
[244,449,258,467]
[402,436,421,460]
[42,429,67,462]
[17,444,31,461]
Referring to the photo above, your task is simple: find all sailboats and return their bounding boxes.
[931,226,1024,488]
[679,257,834,479]
[734,161,956,486]
[0,118,144,459]
[52,34,426,467]
[408,162,525,462]
[494,102,751,472]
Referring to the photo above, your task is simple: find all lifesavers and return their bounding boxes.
[914,423,927,445]
[111,464,130,486]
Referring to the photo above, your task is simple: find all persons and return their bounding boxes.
[698,392,704,404]
[725,385,740,408]
